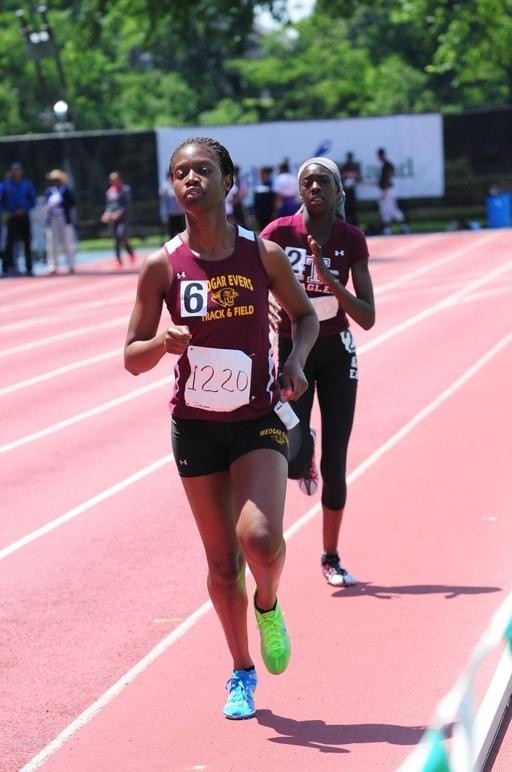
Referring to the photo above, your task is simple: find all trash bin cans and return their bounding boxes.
[485,191,512,228]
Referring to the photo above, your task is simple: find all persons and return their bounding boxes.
[39,170,78,275]
[123,137,321,720]
[0,163,37,277]
[158,149,408,279]
[256,157,375,587]
[99,172,134,268]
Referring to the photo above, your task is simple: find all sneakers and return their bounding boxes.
[253,586,291,675]
[223,665,256,719]
[322,563,355,587]
[298,428,320,496]
